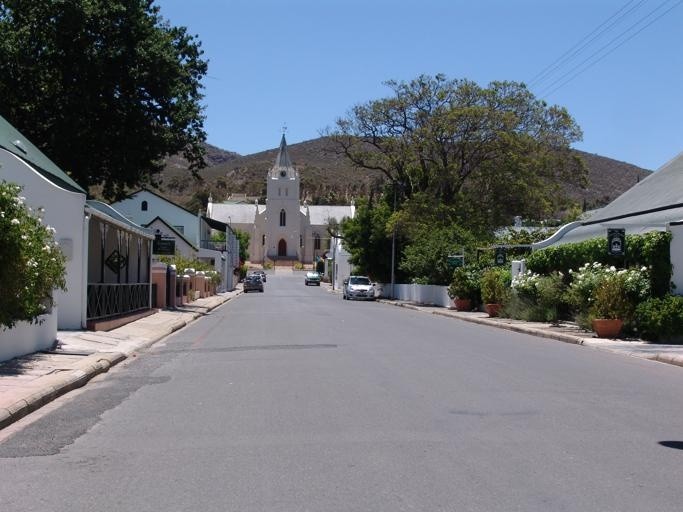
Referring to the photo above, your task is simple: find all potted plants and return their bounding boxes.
[446,263,512,316]
[588,274,628,338]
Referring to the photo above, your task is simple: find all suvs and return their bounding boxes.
[241,273,265,292]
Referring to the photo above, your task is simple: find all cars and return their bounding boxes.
[303,270,321,286]
[342,274,376,300]
[252,271,268,283]
